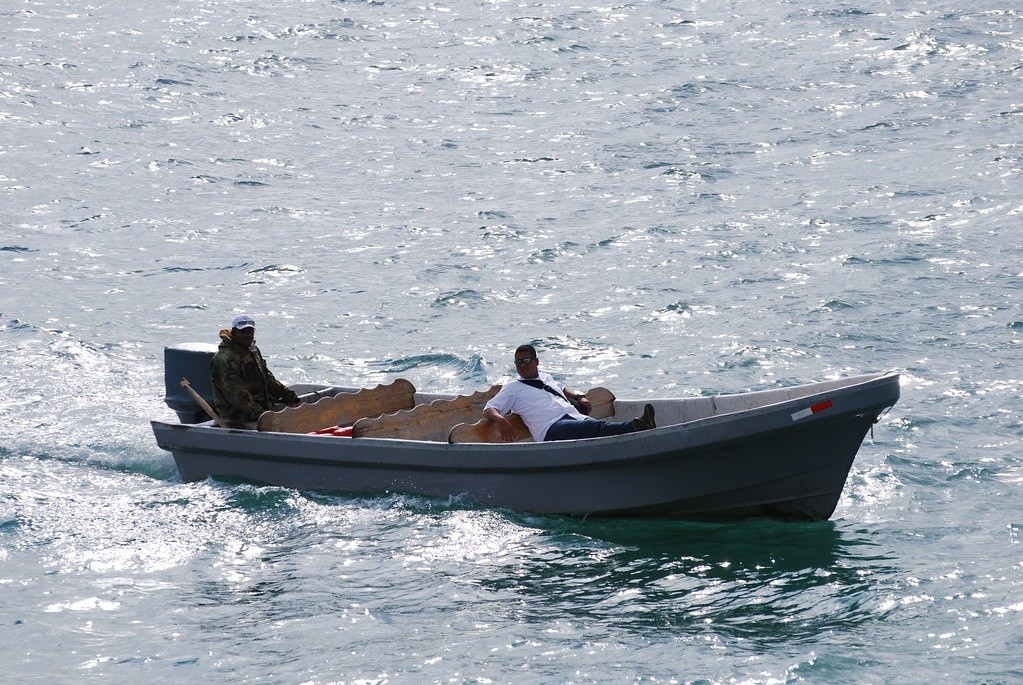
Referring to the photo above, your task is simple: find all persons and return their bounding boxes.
[208,314,300,436]
[482,344,656,444]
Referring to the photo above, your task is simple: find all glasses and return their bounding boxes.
[514,358,536,366]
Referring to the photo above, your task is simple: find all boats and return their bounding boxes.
[153,341,900,522]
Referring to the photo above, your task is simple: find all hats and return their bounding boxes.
[231,315,256,330]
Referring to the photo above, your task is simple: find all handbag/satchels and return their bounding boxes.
[568,395,589,415]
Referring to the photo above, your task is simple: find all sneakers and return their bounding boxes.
[632,417,650,431]
[641,404,656,428]
